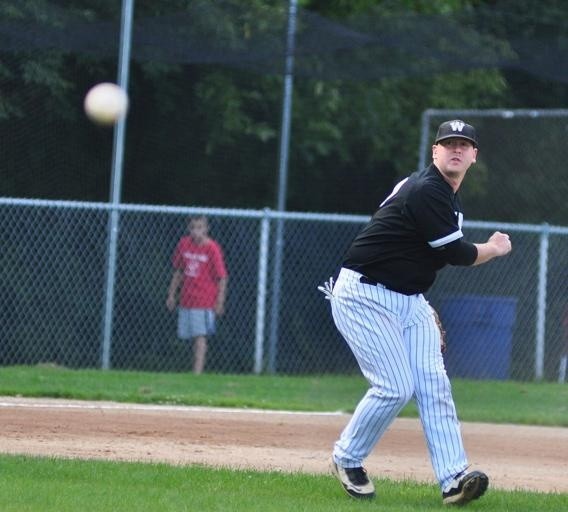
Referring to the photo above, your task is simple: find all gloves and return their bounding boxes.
[317,277,335,300]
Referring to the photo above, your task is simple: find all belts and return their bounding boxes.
[360,276,377,286]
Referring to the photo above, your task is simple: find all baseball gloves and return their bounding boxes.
[427,299,449,356]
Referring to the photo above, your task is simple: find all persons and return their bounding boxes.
[316,117,514,508]
[165,212,229,377]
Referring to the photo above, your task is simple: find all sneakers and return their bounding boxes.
[327,455,376,500]
[442,470,488,507]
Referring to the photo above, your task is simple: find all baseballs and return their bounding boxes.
[85,83,131,124]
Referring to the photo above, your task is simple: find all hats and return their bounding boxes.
[435,120,479,147]
